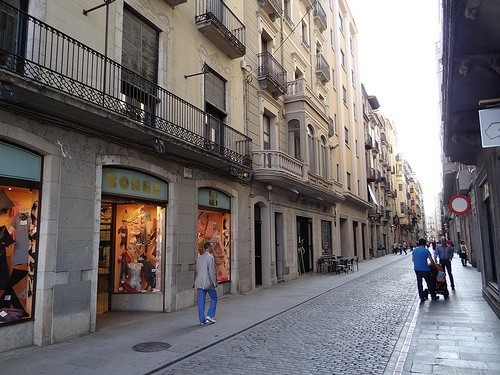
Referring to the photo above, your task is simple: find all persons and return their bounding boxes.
[194,242,218,326]
[396,241,403,255]
[392,242,398,254]
[436,271,446,289]
[434,237,456,291]
[412,238,440,302]
[400,241,408,255]
[447,238,454,247]
[127,259,143,291]
[458,240,468,266]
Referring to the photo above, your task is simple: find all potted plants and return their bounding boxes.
[377,248,381,256]
[381,247,385,256]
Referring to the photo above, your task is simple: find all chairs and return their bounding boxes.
[319,256,359,275]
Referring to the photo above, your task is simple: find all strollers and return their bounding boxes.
[421,263,449,299]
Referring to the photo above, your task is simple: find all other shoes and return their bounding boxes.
[206,316,216,324]
[421,297,427,301]
[200,321,205,326]
[432,296,440,300]
[451,286,456,290]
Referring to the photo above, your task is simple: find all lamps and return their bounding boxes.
[290,188,299,194]
